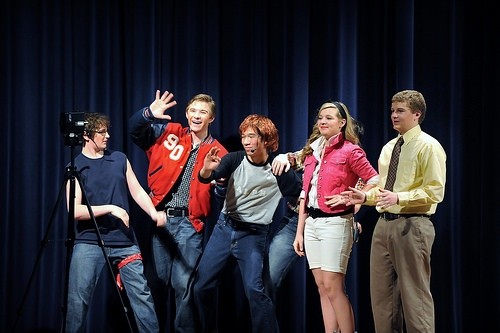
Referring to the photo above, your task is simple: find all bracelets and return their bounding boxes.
[148,107,154,117]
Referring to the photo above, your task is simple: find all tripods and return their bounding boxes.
[11,138,135,333]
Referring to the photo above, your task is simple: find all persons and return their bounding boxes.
[173,114,303,333]
[65,112,167,333]
[266,102,379,333]
[339,90,446,333]
[127,90,228,333]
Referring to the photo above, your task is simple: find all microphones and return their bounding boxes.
[251,148,256,152]
[86,130,107,151]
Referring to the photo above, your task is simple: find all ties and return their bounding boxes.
[383,137,405,192]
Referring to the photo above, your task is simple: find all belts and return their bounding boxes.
[305,205,353,219]
[163,207,189,218]
[379,211,430,222]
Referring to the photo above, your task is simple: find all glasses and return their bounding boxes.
[96,130,105,136]
